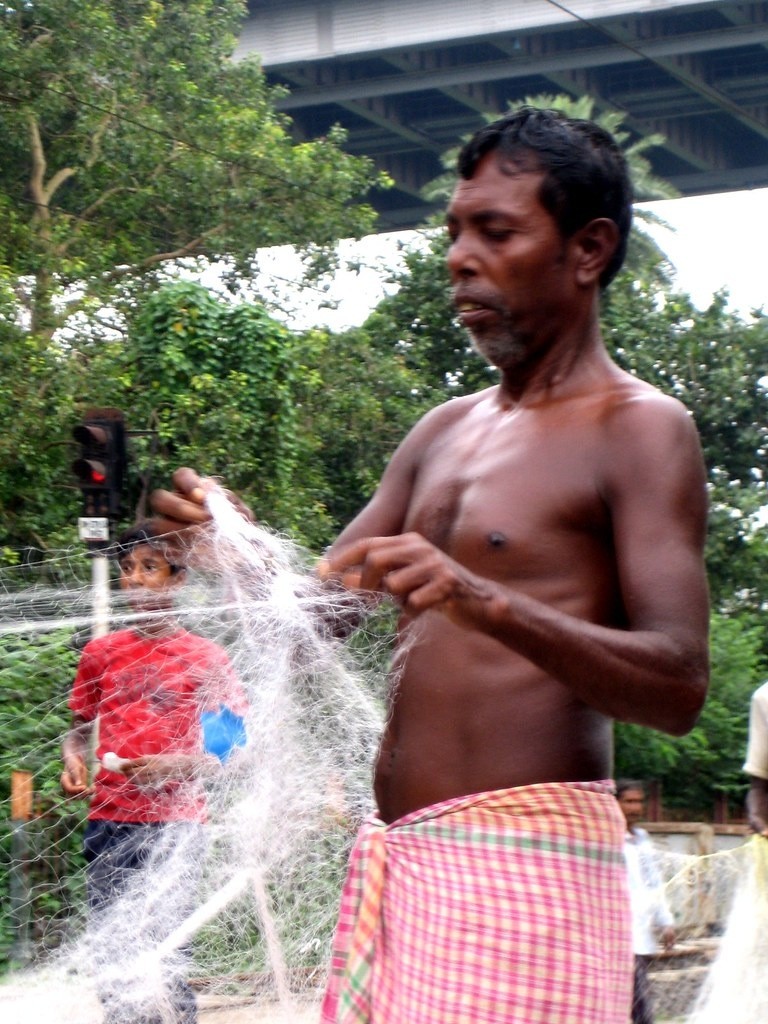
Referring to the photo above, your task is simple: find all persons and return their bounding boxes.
[616,780,677,1024]
[741,683,768,835]
[60,522,251,1024]
[145,105,708,1024]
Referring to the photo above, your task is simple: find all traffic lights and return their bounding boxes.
[66,404,128,526]
